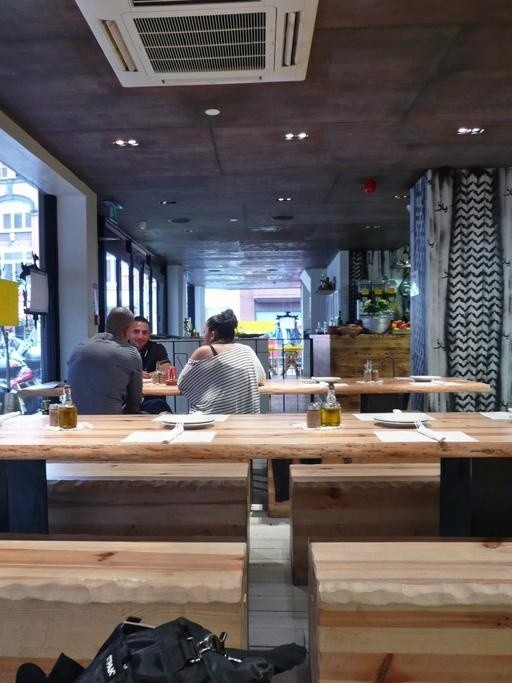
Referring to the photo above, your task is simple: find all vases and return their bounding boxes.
[360,314,392,334]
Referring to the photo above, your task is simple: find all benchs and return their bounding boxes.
[0,538,249,683]
[307,542,512,683]
[45,462,250,542]
[290,463,441,586]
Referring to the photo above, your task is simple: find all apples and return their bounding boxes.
[391,319,407,330]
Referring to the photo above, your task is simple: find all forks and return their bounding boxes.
[414,420,446,442]
[162,420,184,444]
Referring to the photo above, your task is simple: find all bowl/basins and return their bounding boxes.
[337,327,363,335]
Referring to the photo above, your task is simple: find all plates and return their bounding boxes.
[312,376,340,383]
[161,414,216,429]
[372,412,430,428]
[411,375,435,382]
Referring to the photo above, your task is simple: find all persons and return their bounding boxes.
[128,315,173,413]
[177,309,266,413]
[67,305,145,414]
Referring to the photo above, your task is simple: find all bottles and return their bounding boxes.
[153,360,176,385]
[49,385,77,429]
[338,311,342,327]
[364,359,379,381]
[305,383,341,428]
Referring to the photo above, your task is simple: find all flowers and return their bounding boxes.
[358,298,395,320]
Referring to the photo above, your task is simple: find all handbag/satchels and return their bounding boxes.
[73,615,309,681]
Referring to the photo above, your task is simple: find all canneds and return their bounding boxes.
[167,366,176,381]
